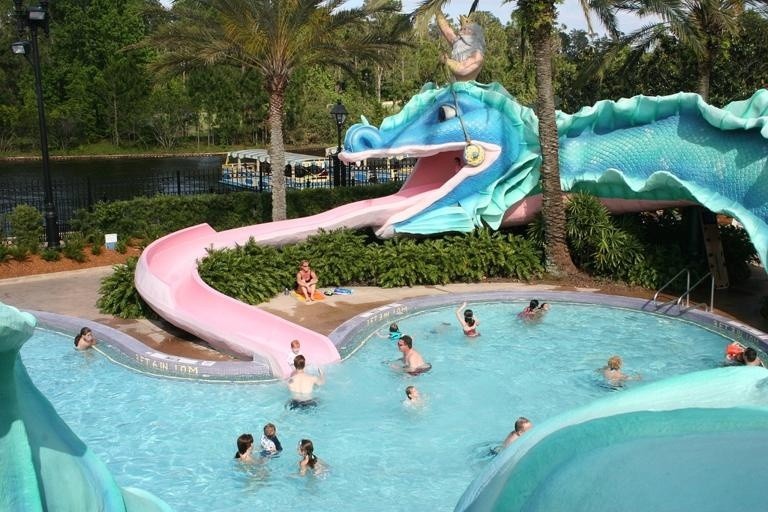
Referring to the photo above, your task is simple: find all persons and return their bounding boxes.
[463,318,481,336]
[288,339,305,369]
[71,326,97,350]
[383,335,432,376]
[297,440,326,474]
[454,157,463,175]
[517,298,539,318]
[234,433,267,464]
[404,385,423,403]
[375,323,415,343]
[261,422,282,452]
[433,6,487,84]
[287,355,325,410]
[529,303,550,322]
[503,416,531,452]
[456,300,479,337]
[295,259,318,302]
[597,355,640,390]
[725,340,762,367]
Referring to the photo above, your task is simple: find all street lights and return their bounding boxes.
[327,97,353,188]
[11,0,64,253]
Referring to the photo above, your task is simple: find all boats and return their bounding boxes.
[344,157,417,185]
[218,148,334,194]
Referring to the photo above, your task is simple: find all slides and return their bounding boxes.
[134,183,443,379]
